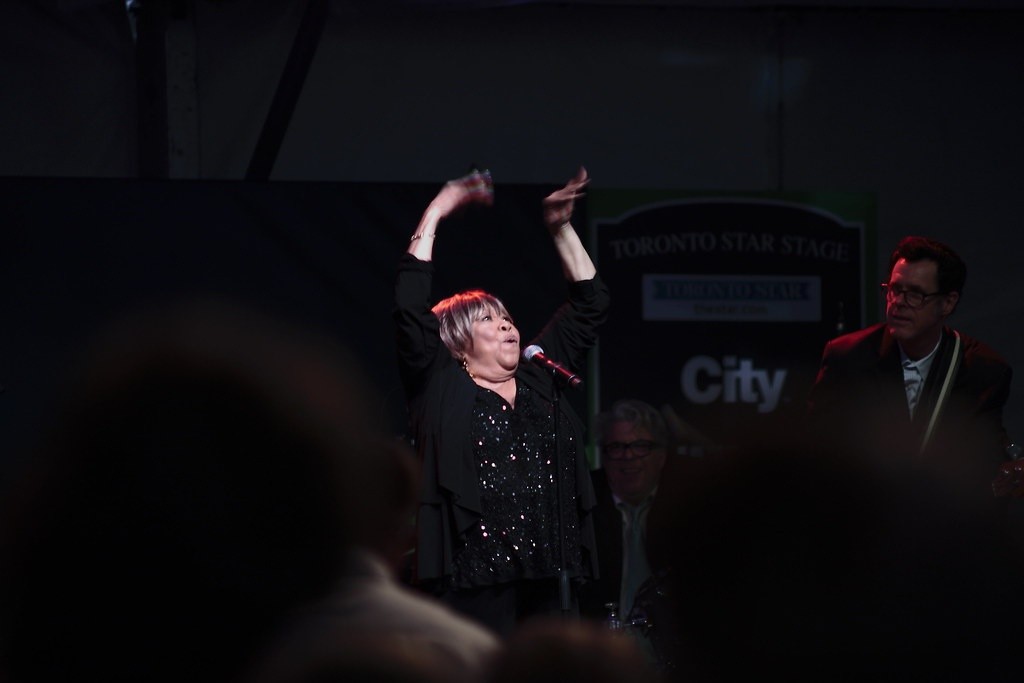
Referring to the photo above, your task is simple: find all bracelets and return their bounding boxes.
[411,233,436,241]
[561,222,569,228]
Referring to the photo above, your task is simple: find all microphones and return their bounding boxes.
[523,345,583,387]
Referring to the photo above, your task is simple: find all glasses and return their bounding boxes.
[881,281,948,309]
[605,438,664,459]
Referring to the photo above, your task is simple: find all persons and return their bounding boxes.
[393,167,612,640]
[803,236,1013,476]
[588,400,676,625]
[0,336,349,682]
[242,436,503,683]
[493,612,659,683]
[646,441,1024,683]
